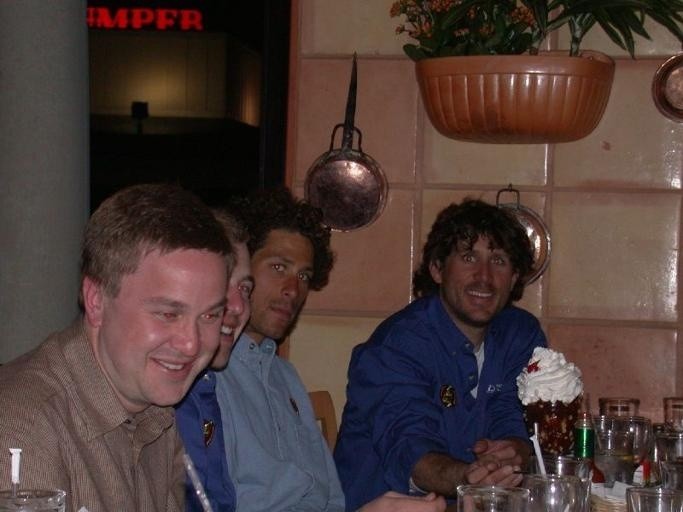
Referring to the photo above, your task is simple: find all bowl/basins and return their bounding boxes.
[651,51,683,122]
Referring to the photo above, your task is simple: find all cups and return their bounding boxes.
[455,396,683,512]
[1,486,66,512]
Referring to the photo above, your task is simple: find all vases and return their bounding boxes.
[415,48,615,143]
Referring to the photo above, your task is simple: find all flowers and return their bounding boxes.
[389,0,683,62]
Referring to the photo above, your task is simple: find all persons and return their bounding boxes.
[212,183,446,512]
[0,180,231,512]
[334,200,558,512]
[173,207,253,510]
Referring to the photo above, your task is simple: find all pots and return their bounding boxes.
[302,53,389,232]
[494,186,552,286]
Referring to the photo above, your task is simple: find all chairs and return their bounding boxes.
[307,390,338,455]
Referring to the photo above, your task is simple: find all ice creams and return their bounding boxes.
[516,347,584,457]
